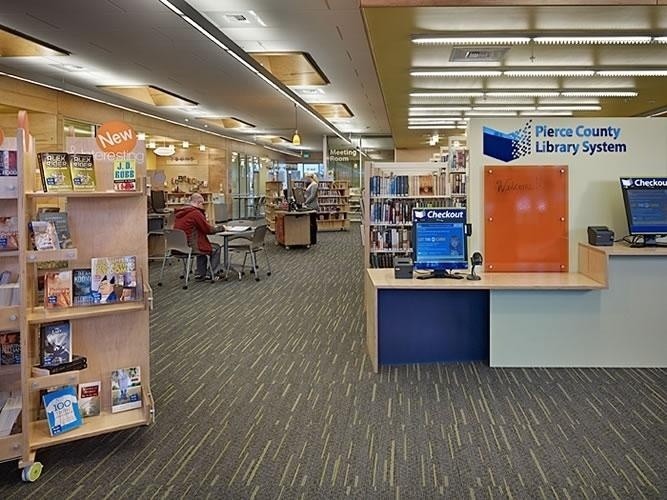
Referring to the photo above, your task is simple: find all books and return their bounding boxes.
[0,264,21,307]
[0,390,22,437]
[318,214,345,220]
[292,182,304,188]
[318,182,346,189]
[0,150,17,176]
[229,225,256,232]
[452,150,466,170]
[67,154,97,192]
[33,256,143,312]
[368,171,469,197]
[370,226,413,249]
[318,206,344,212]
[318,198,347,204]
[31,320,88,377]
[78,381,102,418]
[0,328,21,366]
[441,155,449,170]
[109,366,143,414]
[369,252,415,268]
[34,385,86,437]
[0,216,19,251]
[317,190,341,196]
[369,198,466,225]
[37,152,73,193]
[113,159,137,191]
[32,203,68,250]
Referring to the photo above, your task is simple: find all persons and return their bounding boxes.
[174,192,227,282]
[302,172,320,247]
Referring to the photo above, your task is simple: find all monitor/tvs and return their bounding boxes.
[619,177,667,248]
[411,206,468,280]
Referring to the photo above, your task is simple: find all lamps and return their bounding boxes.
[293,103,300,145]
[154,136,176,156]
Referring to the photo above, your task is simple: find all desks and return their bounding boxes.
[274,210,316,250]
[215,226,255,283]
[364,239,667,374]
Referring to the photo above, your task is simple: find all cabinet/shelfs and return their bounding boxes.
[265,181,282,233]
[166,192,214,228]
[363,160,466,273]
[166,159,198,166]
[291,179,350,232]
[0,110,155,483]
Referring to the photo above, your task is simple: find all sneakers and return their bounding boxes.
[193,275,205,282]
[205,275,219,283]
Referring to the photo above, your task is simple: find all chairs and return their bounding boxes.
[228,223,271,281]
[148,232,191,288]
[157,227,215,290]
